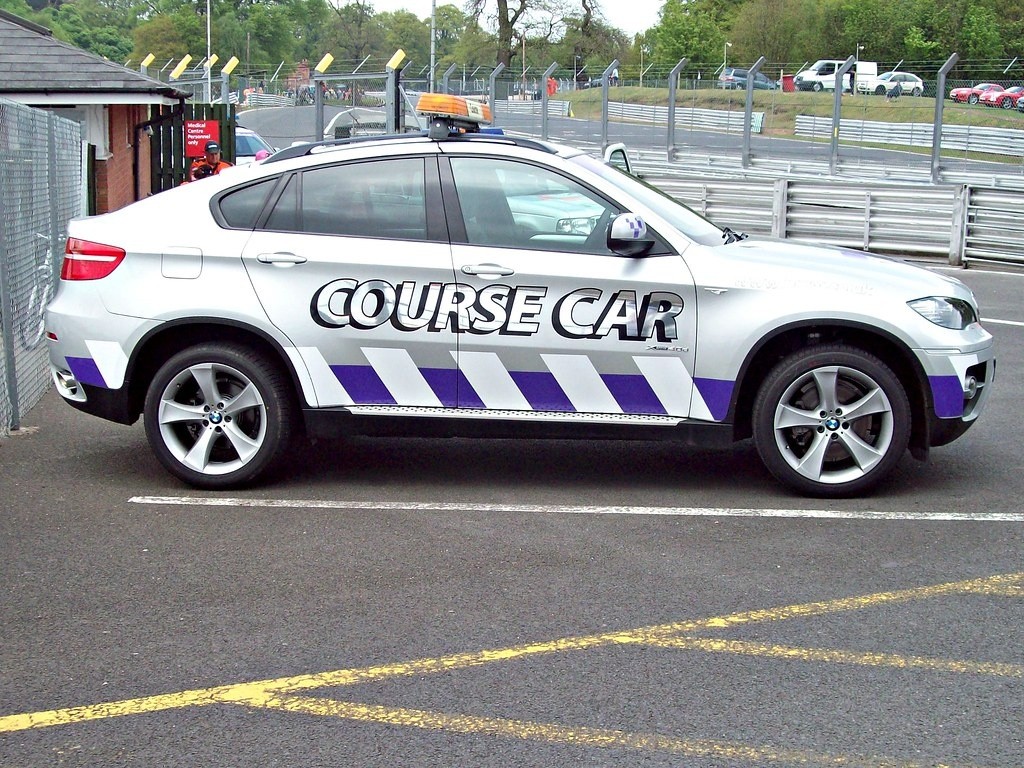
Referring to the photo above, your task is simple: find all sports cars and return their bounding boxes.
[950,83,1004,106]
[979,86,1024,109]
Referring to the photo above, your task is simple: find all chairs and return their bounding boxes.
[884,89,905,102]
[477,188,521,246]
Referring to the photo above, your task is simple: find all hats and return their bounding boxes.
[204,140,220,154]
[256,150,269,160]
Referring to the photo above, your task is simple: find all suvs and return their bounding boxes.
[857,71,924,97]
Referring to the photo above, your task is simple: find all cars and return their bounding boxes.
[1017,97,1024,111]
[584,78,604,88]
[291,85,315,98]
[229,121,279,166]
[40,92,998,498]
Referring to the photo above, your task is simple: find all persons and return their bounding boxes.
[190,141,232,181]
[547,77,557,97]
[611,68,618,81]
[180,164,213,185]
[258,81,265,93]
[287,86,314,105]
[884,81,902,102]
[850,66,856,96]
[324,86,352,100]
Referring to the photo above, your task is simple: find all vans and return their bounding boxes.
[718,68,780,91]
[794,60,878,92]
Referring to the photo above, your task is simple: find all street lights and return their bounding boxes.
[573,55,581,89]
[640,47,649,85]
[196,7,211,103]
[724,42,732,89]
[854,43,864,95]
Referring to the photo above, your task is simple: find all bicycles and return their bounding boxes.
[20,226,65,352]
